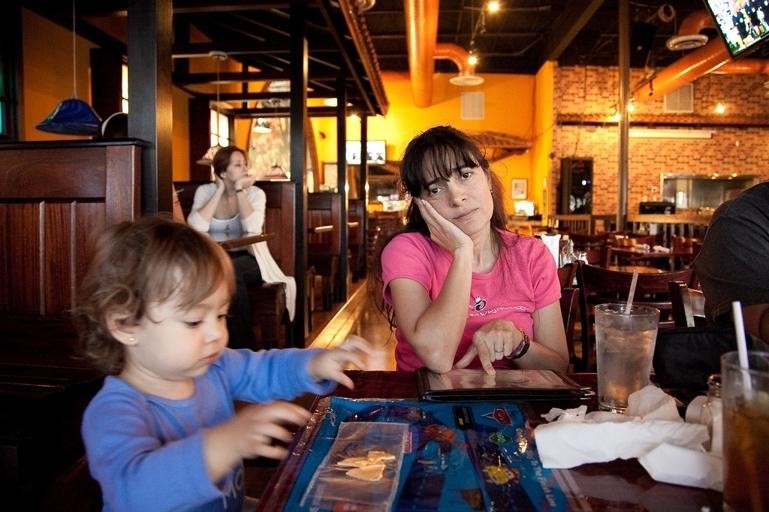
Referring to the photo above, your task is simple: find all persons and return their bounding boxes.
[378,127,571,379]
[690,179,768,344]
[72,213,374,512]
[186,146,297,347]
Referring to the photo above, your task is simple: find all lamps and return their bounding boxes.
[466,1,478,67]
[34,0,101,136]
[194,50,229,169]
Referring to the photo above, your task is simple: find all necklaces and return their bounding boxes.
[221,186,234,237]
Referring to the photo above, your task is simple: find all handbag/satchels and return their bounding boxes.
[649,325,756,405]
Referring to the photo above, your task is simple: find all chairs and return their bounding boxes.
[16,373,106,511]
[501,214,708,372]
[172,180,367,348]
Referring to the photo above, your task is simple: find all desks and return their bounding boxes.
[255,370,769,512]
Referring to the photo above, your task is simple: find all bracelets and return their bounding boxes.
[234,189,244,194]
[510,335,531,359]
[512,328,525,355]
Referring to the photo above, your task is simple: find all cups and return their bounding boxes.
[649,224,658,235]
[594,303,662,412]
[720,350,768,511]
[675,192,687,208]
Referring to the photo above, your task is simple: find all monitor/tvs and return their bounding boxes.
[346,140,387,166]
[706,0,769,60]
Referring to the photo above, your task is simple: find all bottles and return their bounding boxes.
[699,374,722,452]
